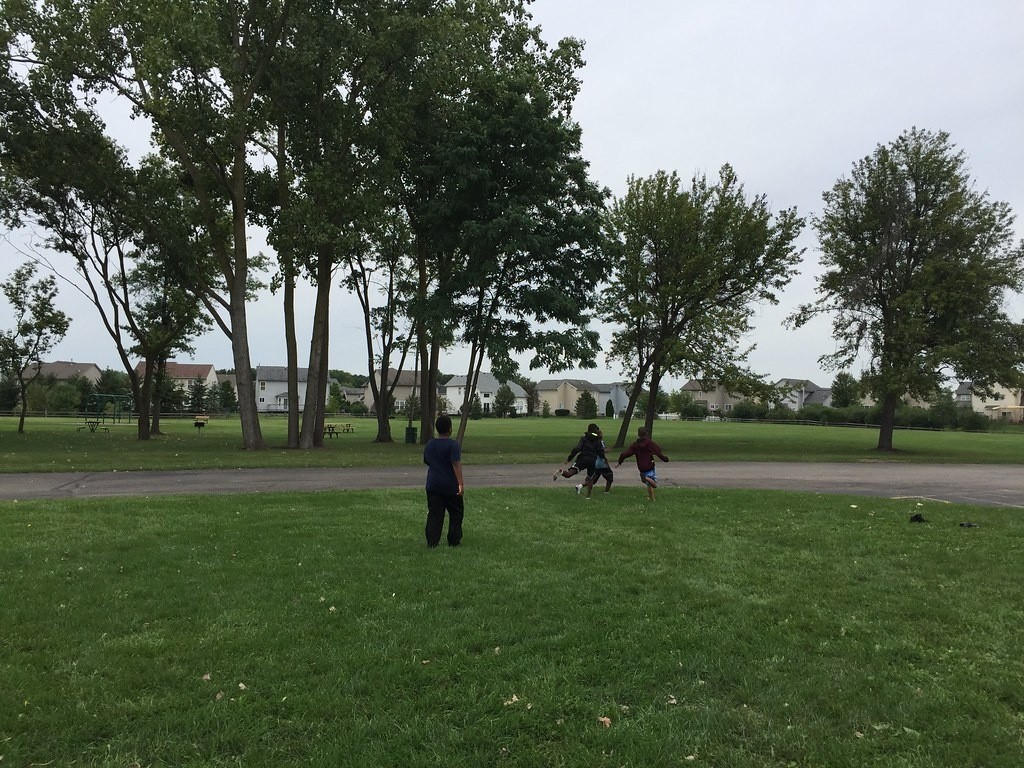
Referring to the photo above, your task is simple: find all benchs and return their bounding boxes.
[100,427,110,433]
[77,426,85,432]
[324,423,357,438]
[193,416,210,424]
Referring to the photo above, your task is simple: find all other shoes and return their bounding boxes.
[552,468,563,481]
[575,484,583,495]
[586,497,591,500]
[640,473,647,483]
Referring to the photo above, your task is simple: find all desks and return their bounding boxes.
[85,421,101,433]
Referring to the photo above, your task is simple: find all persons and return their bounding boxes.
[553,423,614,499]
[424,416,464,549]
[615,426,670,501]
